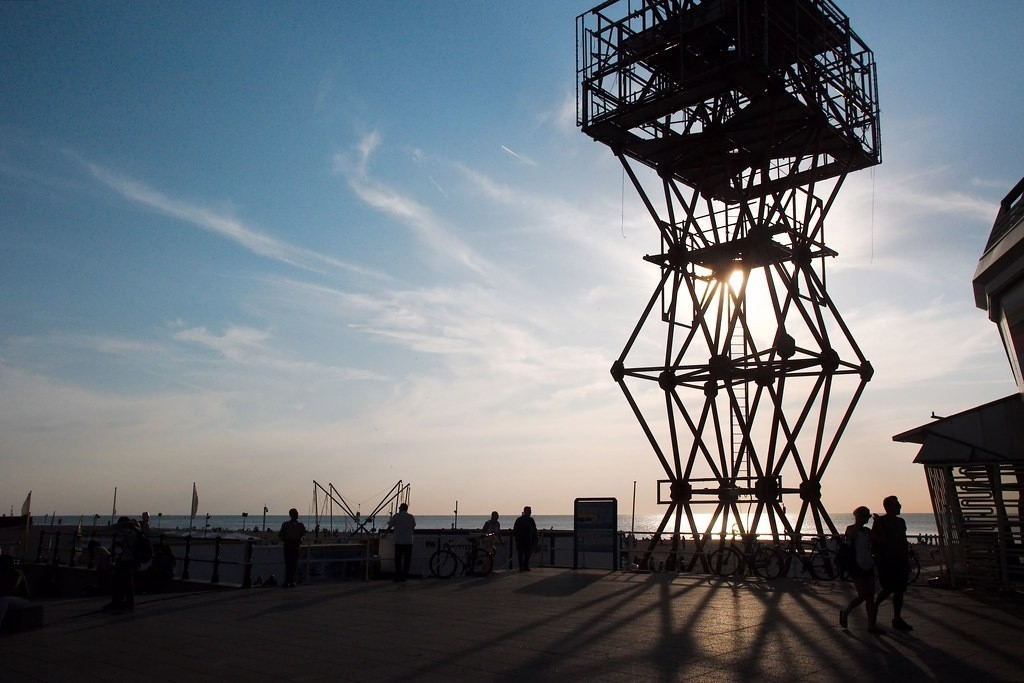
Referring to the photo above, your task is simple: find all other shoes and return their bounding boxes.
[292,581,297,587]
[866,603,878,623]
[283,583,290,587]
[892,617,913,630]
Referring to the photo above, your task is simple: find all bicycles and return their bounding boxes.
[773,530,841,582]
[429,536,493,579]
[906,553,921,584]
[709,523,784,580]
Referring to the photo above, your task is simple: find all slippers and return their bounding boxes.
[840,610,847,628]
[868,627,886,634]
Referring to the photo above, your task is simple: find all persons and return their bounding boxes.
[278,509,305,588]
[839,496,914,636]
[86,516,173,611]
[482,513,504,569]
[385,503,416,581]
[514,507,539,571]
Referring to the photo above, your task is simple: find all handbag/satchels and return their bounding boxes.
[834,544,859,576]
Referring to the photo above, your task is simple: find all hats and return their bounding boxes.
[853,507,872,518]
